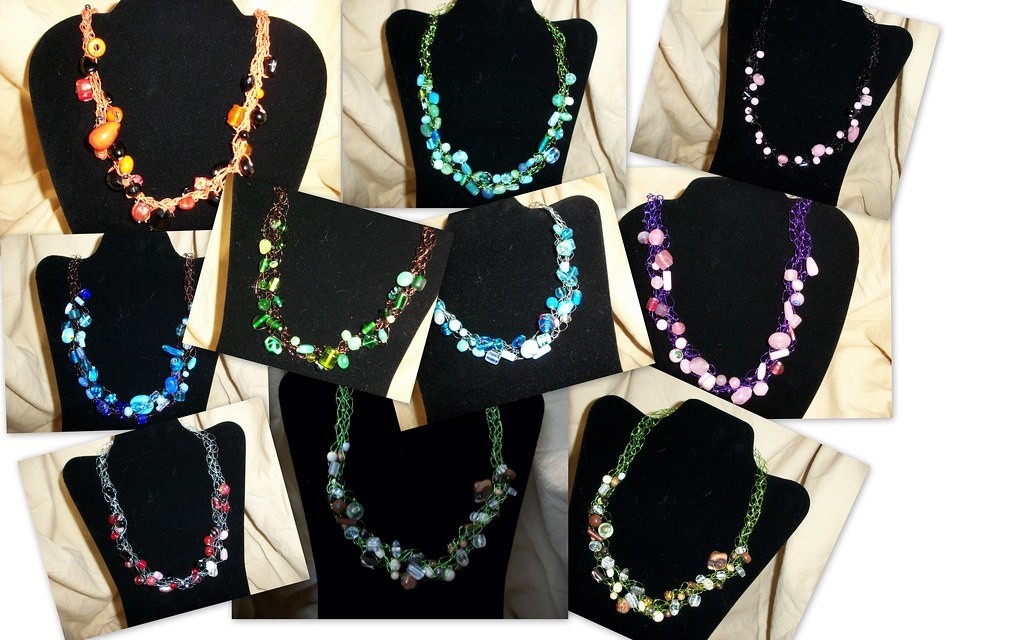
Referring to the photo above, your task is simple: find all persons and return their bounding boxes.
[253,185,437,371]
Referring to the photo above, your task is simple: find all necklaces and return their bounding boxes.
[69,254,196,424]
[588,403,769,622]
[743,1,879,167]
[434,206,581,365]
[77,2,278,223]
[326,385,516,590]
[96,424,229,593]
[637,195,820,405]
[422,1,578,198]
